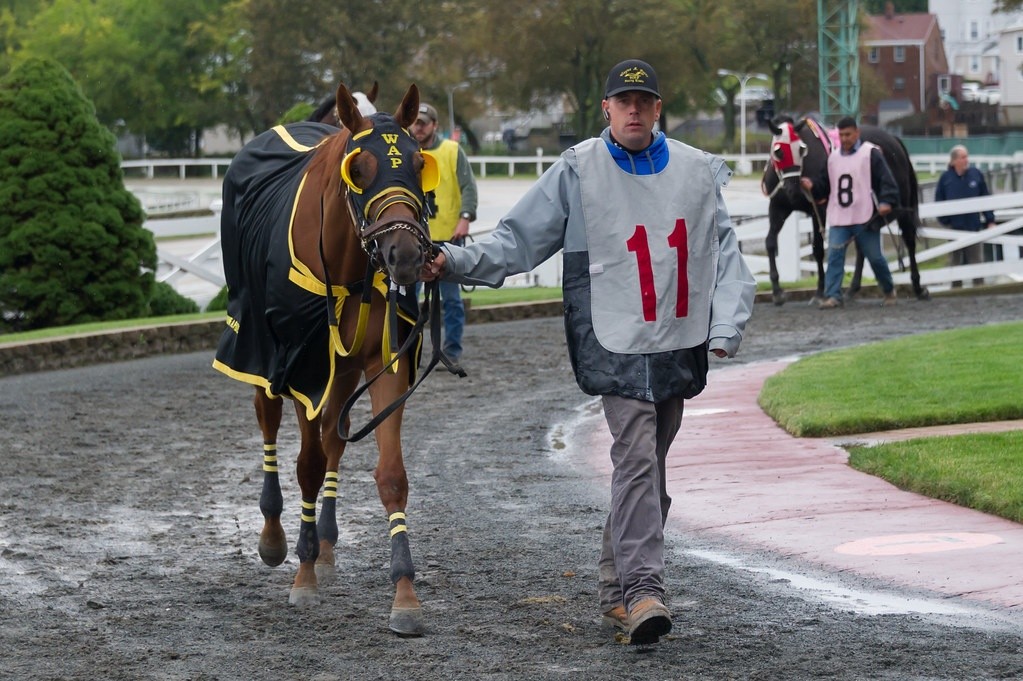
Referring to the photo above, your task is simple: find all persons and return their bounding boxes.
[934,145,998,289]
[800,116,899,311]
[425,60,758,649]
[400,103,480,371]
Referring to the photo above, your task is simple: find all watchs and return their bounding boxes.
[459,212,472,220]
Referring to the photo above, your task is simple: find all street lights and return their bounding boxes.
[719,70,767,176]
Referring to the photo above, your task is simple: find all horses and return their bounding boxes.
[764,115,930,307]
[221,80,433,635]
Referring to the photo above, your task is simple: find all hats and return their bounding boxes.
[605,59,661,99]
[419,103,437,124]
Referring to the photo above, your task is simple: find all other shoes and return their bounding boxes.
[444,351,459,368]
[882,293,894,307]
[626,597,673,648]
[602,604,633,633]
[819,296,843,308]
